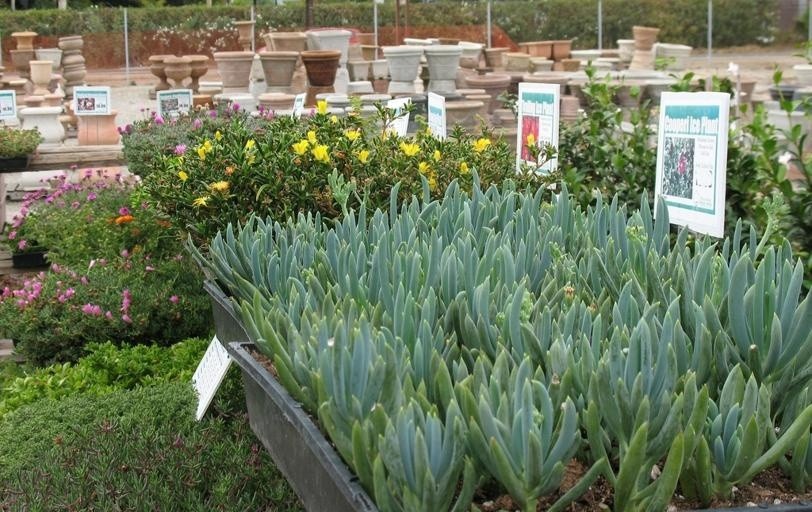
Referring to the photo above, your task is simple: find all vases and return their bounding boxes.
[0,16,811,152]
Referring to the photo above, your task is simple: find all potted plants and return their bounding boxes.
[0,120,43,170]
